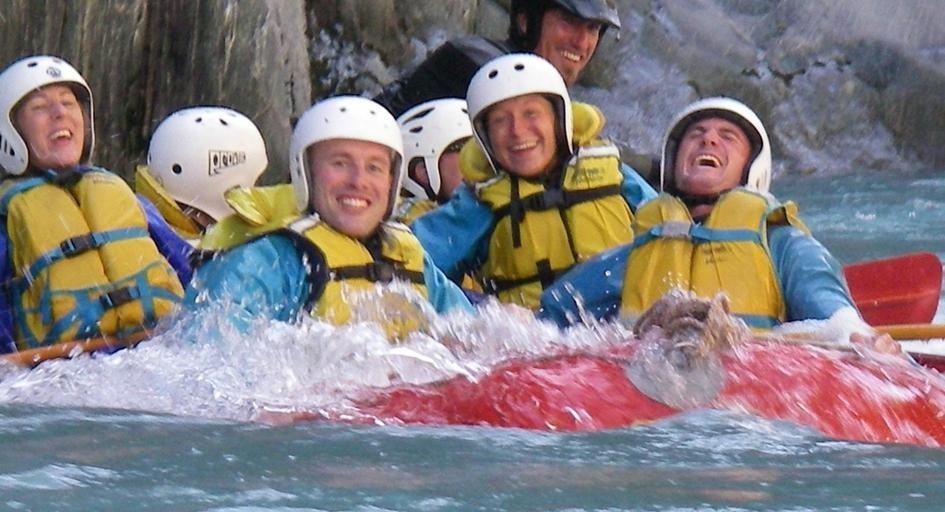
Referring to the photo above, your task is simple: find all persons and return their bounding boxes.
[154,96,535,357]
[1,54,187,373]
[133,106,270,286]
[537,95,916,365]
[409,51,656,324]
[388,99,484,304]
[375,0,623,117]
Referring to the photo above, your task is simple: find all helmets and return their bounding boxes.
[466,52,573,174]
[660,96,772,194]
[511,0,620,36]
[0,55,95,175]
[146,106,268,222]
[395,98,473,203]
[288,96,405,224]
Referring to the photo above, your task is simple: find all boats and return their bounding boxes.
[194,194,945,448]
